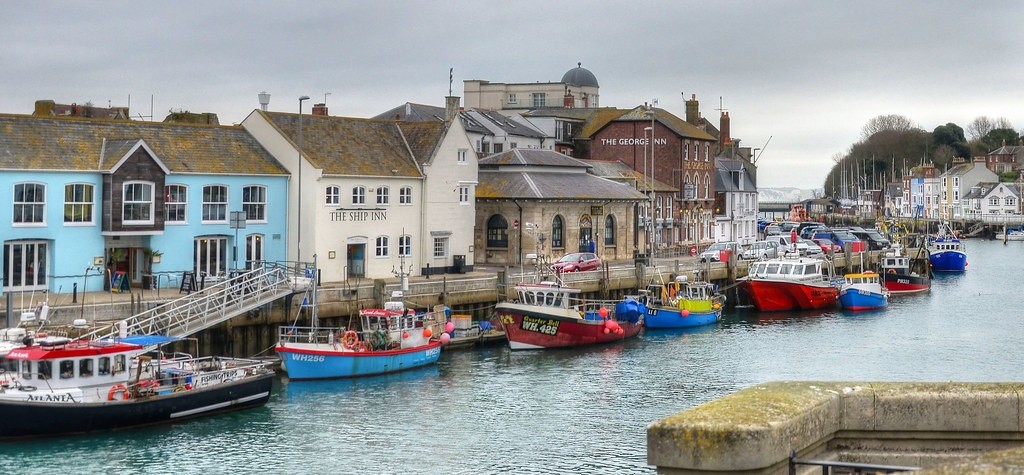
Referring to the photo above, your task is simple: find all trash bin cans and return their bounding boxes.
[454,255,466,274]
[635,253,649,267]
[231,271,253,295]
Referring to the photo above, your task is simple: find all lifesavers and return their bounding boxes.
[35,333,49,338]
[108,384,128,399]
[888,269,897,274]
[343,330,359,350]
[864,272,875,274]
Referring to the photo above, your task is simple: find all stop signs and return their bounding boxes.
[514,220,519,226]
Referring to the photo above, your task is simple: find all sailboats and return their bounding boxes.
[832,153,968,294]
[995,180,1024,241]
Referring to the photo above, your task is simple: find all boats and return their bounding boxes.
[273,227,454,380]
[494,254,646,352]
[640,258,727,328]
[736,230,843,313]
[0,264,277,432]
[836,250,891,313]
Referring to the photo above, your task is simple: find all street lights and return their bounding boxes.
[753,148,761,165]
[644,111,656,265]
[324,92,333,115]
[642,126,654,257]
[730,136,741,242]
[296,95,311,276]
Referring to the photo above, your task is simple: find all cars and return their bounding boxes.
[549,252,602,273]
[743,218,891,260]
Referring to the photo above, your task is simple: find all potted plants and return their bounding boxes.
[151,250,163,262]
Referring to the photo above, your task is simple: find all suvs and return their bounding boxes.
[698,241,744,264]
